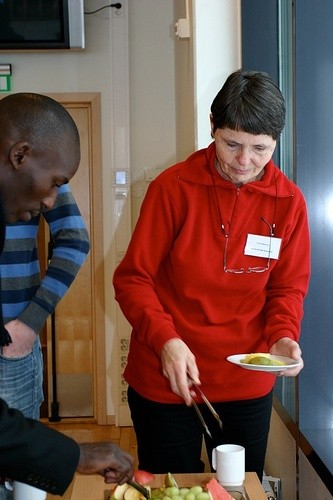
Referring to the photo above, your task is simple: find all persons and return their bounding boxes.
[112,72,309,475]
[0,92,137,500]
[1,182,88,500]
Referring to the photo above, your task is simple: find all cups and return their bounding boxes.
[212,445,245,486]
[5,481,46,500]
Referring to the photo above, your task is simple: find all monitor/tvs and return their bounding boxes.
[0,0,85,50]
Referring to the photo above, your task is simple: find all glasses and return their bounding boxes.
[221,217,273,274]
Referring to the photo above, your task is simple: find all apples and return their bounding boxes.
[109,469,155,500]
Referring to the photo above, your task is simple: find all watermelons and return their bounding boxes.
[206,478,235,500]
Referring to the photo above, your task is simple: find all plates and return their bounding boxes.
[227,354,301,371]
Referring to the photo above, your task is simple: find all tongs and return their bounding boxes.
[108,470,150,500]
[185,371,223,437]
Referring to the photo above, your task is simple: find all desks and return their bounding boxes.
[45,472,268,500]
